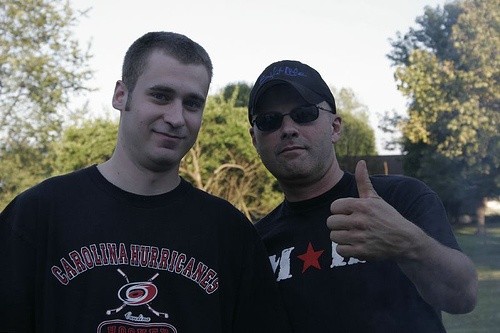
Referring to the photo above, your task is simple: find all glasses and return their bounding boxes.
[254,104,335,132]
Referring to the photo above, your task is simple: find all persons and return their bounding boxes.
[246,60,478,333]
[0,32,291,333]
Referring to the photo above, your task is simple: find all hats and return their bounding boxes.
[248,60,335,127]
